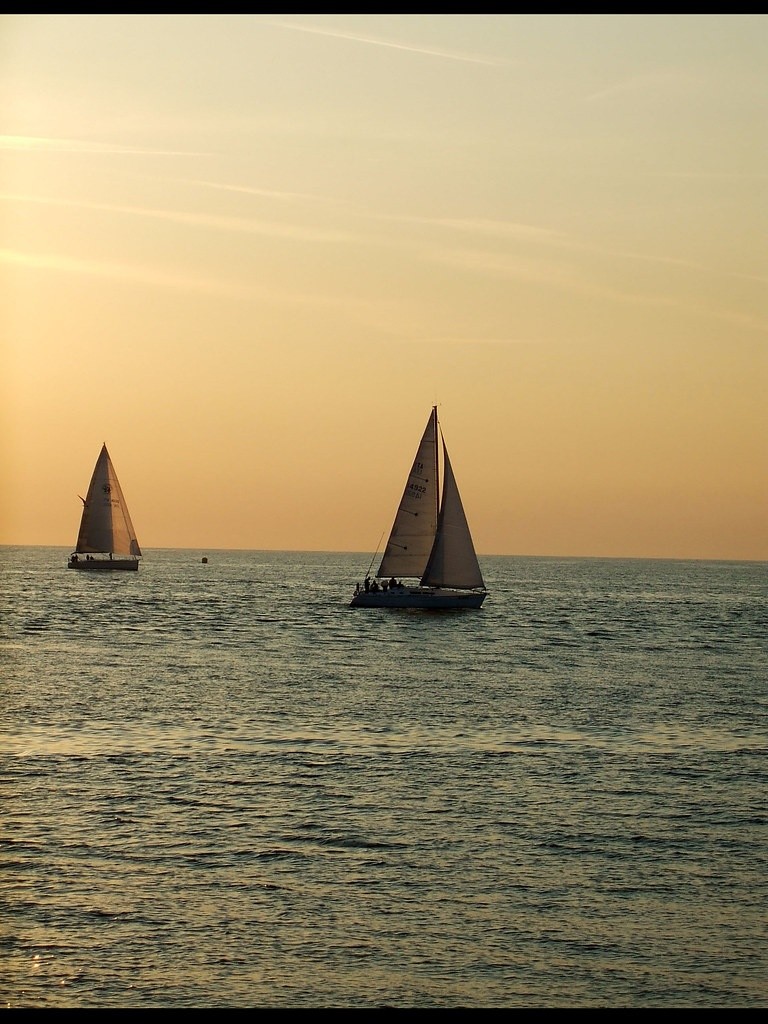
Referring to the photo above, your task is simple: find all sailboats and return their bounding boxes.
[67,444,142,572]
[347,405,491,612]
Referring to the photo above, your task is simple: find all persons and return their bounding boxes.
[365,577,405,593]
[71,555,78,563]
[86,555,93,561]
